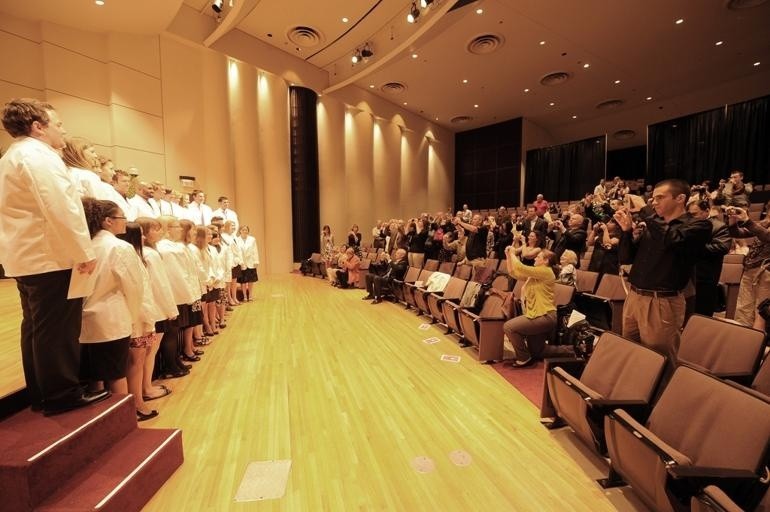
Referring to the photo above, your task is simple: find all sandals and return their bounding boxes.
[135,296,255,422]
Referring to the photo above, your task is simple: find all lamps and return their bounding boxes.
[407,0,436,26]
[211,1,225,14]
[351,42,373,64]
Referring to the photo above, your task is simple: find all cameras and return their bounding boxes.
[623,197,631,210]
[726,207,737,215]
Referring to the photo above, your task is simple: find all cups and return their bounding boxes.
[415,280,423,288]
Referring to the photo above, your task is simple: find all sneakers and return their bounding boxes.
[362,293,382,304]
[331,282,349,288]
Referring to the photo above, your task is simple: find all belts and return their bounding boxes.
[630,285,682,298]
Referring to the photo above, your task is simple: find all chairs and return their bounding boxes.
[719,254,745,319]
[748,185,770,222]
[539,314,769,512]
[310,239,629,363]
[470,203,533,223]
[547,201,580,221]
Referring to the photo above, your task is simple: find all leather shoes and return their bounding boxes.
[31,381,112,416]
[512,358,534,368]
